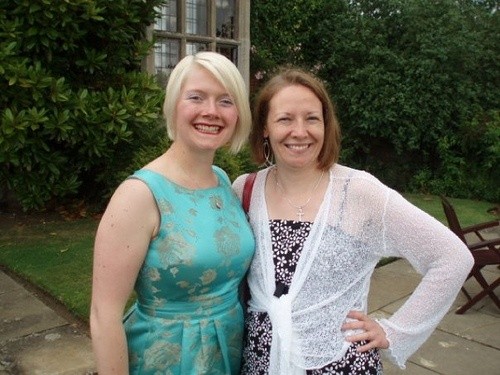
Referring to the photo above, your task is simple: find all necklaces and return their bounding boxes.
[274,161,325,222]
[170,150,223,209]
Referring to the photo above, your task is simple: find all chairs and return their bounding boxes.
[440,194,500,315]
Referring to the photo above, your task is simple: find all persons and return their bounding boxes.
[233,69,475,375]
[89,51,256,375]
